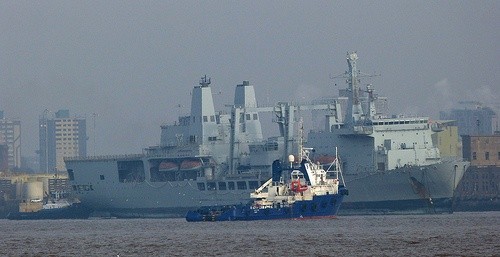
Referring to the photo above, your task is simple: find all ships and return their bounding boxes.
[64,51,472,216]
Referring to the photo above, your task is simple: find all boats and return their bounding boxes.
[184,118,349,221]
[5,191,91,221]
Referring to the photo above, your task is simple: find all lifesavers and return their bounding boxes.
[291,180,301,191]
